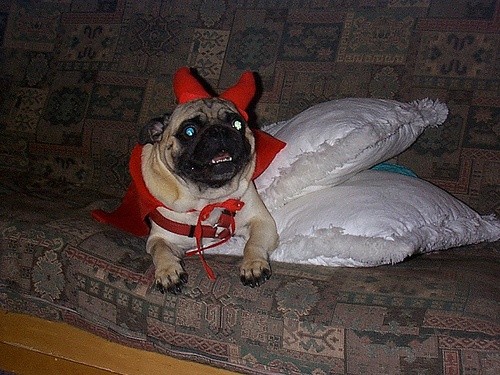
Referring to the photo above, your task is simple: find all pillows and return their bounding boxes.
[189,96,500,269]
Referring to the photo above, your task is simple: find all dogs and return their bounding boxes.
[138,66,279,295]
